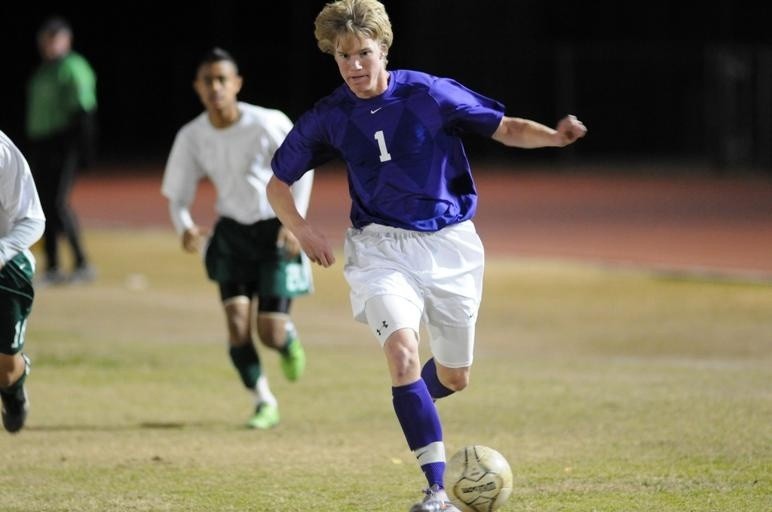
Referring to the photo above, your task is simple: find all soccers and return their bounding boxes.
[442,445,514,512]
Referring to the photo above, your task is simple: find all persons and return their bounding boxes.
[1,130,47,434]
[266,0,592,511]
[22,15,99,286]
[157,44,314,431]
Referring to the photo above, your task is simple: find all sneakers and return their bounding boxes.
[247,402,280,430]
[410,484,451,511]
[1,385,26,432]
[279,320,304,381]
